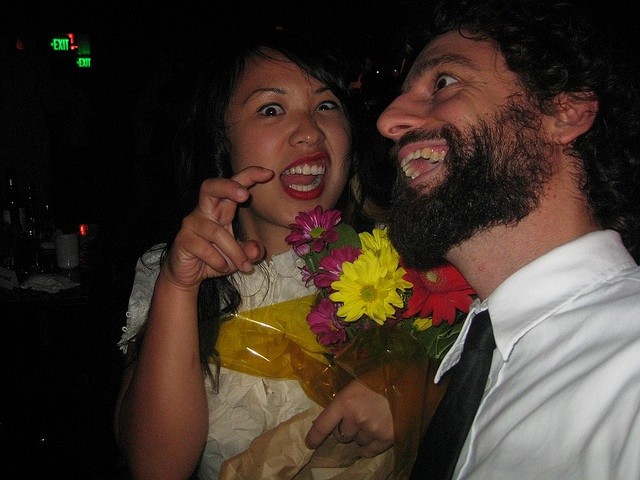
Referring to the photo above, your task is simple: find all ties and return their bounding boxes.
[408,310,497,479]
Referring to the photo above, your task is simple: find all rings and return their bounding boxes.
[338,421,352,443]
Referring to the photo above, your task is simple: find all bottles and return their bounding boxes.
[0,178,46,289]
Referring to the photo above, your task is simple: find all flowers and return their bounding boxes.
[284,204,477,390]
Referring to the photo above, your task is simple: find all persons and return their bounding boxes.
[113,33,444,480]
[376,0,638,480]
